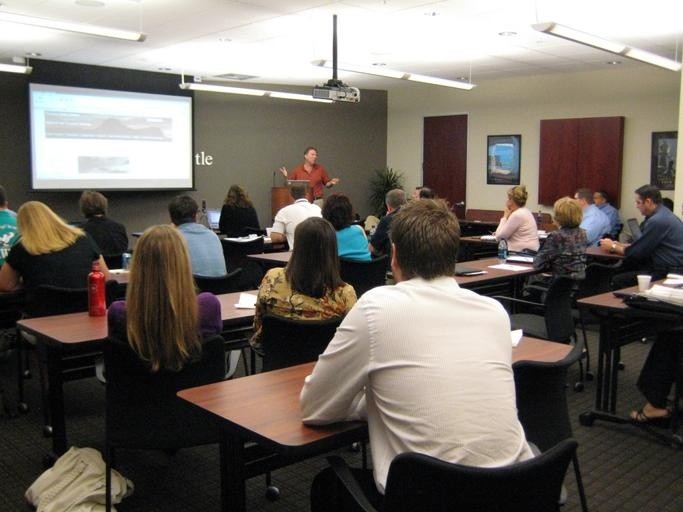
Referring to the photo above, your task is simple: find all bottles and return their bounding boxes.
[497,238,507,263]
[369,225,376,241]
[537,211,542,229]
[87,262,106,317]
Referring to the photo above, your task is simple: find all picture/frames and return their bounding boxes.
[649,131,679,191]
[486,134,522,185]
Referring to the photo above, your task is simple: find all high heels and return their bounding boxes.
[628,408,672,429]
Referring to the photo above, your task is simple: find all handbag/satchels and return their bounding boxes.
[23,445,135,512]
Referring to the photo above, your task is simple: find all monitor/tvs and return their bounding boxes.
[286,179,311,188]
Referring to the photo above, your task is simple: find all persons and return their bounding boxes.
[0,188,130,294]
[279,147,340,206]
[106,224,223,381]
[572,185,683,285]
[270,183,323,251]
[299,194,538,512]
[168,195,227,277]
[530,196,587,304]
[219,184,261,237]
[321,193,371,263]
[493,187,540,256]
[368,187,432,259]
[627,320,682,433]
[251,218,358,356]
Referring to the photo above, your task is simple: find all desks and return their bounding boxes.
[104,268,132,285]
[579,278,683,446]
[16,290,259,466]
[244,249,294,267]
[176,328,589,512]
[388,255,548,314]
[460,230,549,248]
[584,244,630,259]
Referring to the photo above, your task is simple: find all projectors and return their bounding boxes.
[310,79,363,105]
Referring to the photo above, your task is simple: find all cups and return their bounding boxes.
[636,275,652,292]
[266,228,272,237]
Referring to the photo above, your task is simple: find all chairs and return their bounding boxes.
[17,279,120,438]
[530,258,625,379]
[340,254,388,299]
[220,238,264,276]
[260,312,345,376]
[493,275,575,390]
[191,268,243,295]
[324,438,578,511]
[103,334,224,512]
[512,339,590,507]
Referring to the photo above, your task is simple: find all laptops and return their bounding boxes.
[625,217,640,238]
[207,207,223,230]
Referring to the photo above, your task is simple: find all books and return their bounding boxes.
[455,268,488,276]
[506,255,533,264]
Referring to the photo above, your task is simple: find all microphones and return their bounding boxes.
[271,170,277,187]
